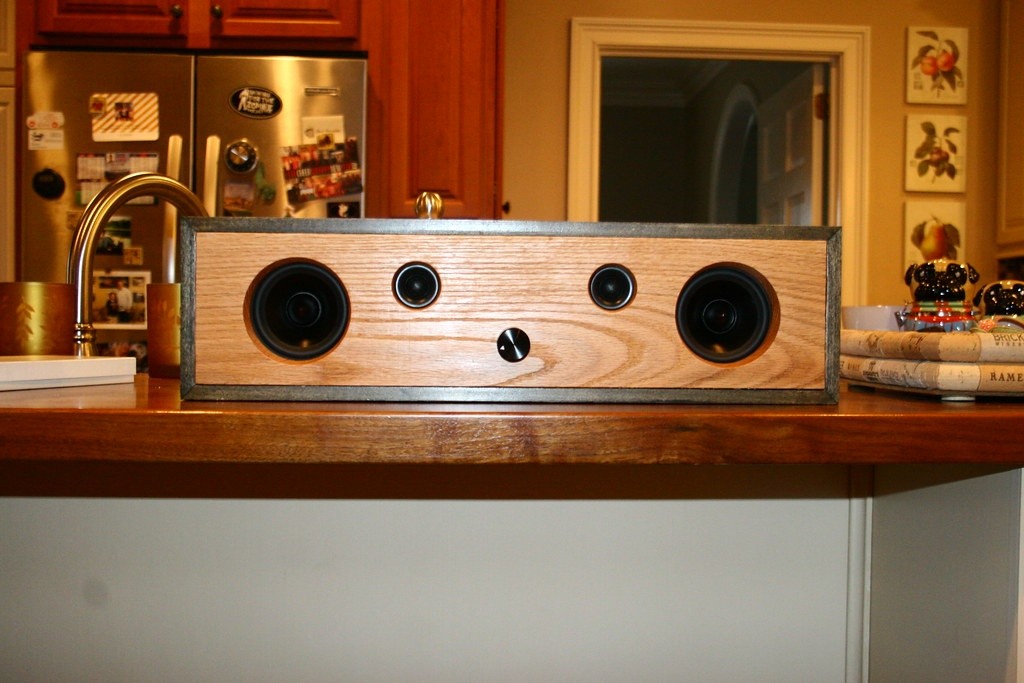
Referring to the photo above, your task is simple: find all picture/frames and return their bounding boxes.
[907,25,970,105]
[903,201,966,277]
[906,112,967,195]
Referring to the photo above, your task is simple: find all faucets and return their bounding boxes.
[64,170,211,356]
